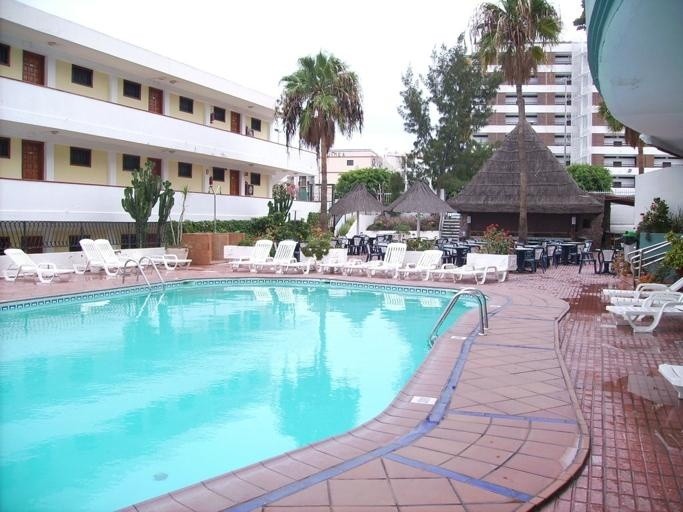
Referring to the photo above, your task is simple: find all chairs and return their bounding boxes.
[598,269,682,421]
[225,235,621,288]
[1,238,191,284]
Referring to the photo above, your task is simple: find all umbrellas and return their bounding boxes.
[382,181,457,239]
[328,182,385,234]
